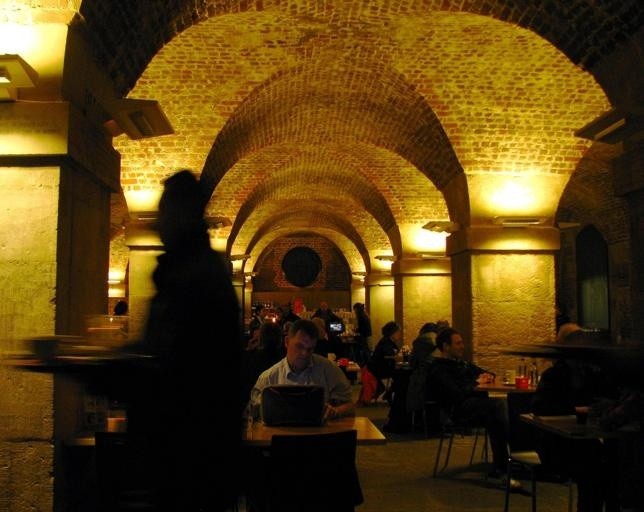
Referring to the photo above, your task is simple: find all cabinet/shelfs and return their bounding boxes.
[332,309,356,339]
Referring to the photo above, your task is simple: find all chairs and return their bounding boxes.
[268,430,356,512]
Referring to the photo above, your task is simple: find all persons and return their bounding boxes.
[532,321,613,417]
[245,301,449,422]
[430,328,515,474]
[57,170,243,512]
[113,297,129,315]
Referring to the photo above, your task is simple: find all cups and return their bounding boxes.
[515,377,529,389]
[503,370,516,386]
[87,328,120,346]
[403,351,408,364]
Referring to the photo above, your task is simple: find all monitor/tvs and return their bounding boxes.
[327,322,345,335]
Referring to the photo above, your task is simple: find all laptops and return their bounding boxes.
[262,385,327,428]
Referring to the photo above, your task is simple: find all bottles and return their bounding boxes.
[530,362,538,386]
[253,299,274,308]
[518,358,526,377]
